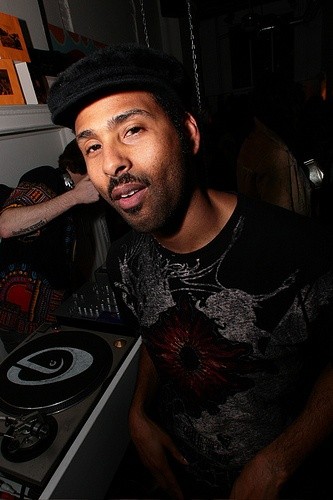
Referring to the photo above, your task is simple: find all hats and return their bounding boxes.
[47,44,190,130]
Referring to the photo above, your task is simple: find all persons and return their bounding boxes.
[0,38,333,500]
